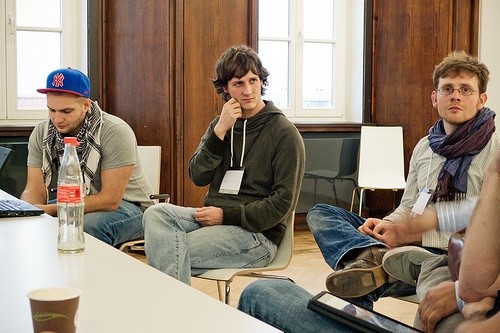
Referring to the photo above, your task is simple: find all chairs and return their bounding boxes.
[299,138,361,208]
[351,125,406,217]
[116,146,169,253]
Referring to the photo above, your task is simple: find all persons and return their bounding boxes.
[141,45,308,287]
[236,49,499,333]
[20,67,156,248]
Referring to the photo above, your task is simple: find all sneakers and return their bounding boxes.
[382,246,439,286]
[326,245,399,297]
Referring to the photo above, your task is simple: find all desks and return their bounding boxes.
[0,188,287,332]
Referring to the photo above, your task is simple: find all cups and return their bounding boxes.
[27,287,80,333]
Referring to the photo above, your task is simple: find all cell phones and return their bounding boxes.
[312,291,427,333]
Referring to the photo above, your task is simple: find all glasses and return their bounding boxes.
[436,86,482,96]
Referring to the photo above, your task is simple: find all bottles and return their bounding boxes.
[57,137,85,252]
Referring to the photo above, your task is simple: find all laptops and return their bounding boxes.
[0,146,45,219]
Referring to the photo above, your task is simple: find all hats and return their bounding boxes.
[37,66,90,98]
